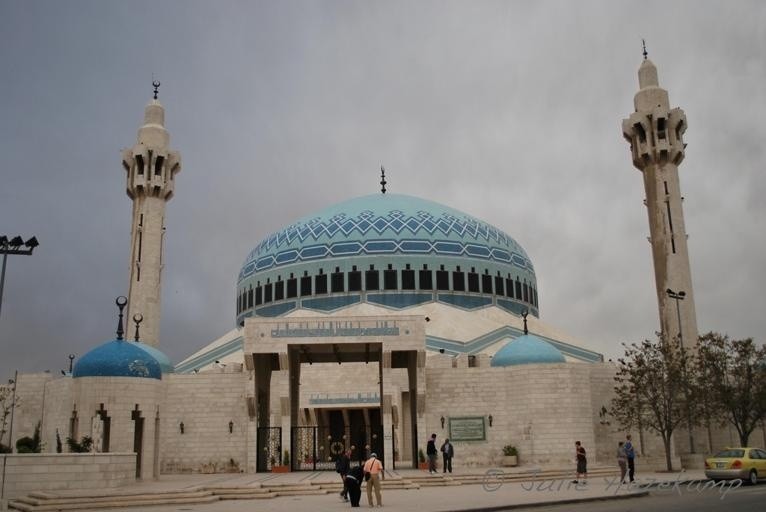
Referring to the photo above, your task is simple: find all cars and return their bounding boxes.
[703,446,766,484]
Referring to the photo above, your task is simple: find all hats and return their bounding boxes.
[371,453,377,458]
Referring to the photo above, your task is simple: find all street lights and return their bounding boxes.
[0,235,40,318]
[665,288,696,456]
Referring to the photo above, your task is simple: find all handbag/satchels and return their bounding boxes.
[336,459,341,473]
[365,472,370,481]
[617,457,625,464]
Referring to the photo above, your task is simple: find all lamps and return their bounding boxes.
[488,414,493,426]
[228,419,234,434]
[440,415,444,428]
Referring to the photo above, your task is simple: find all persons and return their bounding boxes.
[624,434,640,484]
[441,438,454,473]
[616,441,628,484]
[343,463,370,507]
[363,453,385,508]
[427,433,440,474]
[340,448,352,501]
[571,440,588,484]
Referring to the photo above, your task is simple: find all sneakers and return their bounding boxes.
[573,480,579,483]
[369,504,383,508]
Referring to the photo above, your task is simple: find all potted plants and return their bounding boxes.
[502,443,520,467]
[418,447,441,470]
[179,421,184,434]
[270,448,290,472]
